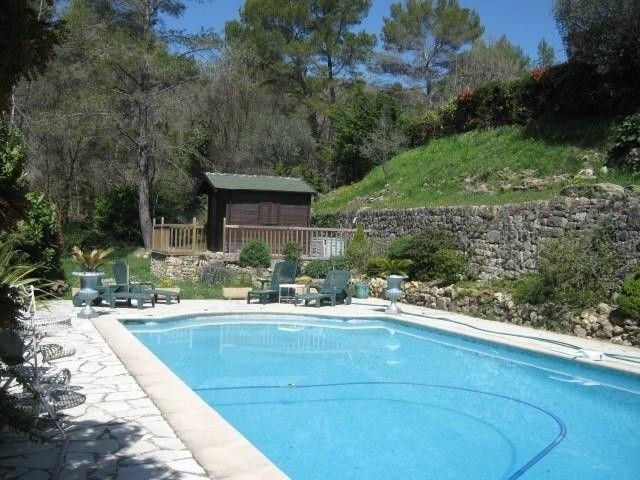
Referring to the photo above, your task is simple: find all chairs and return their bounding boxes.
[94,259,182,309]
[0,282,86,442]
[297,268,353,309]
[246,259,298,305]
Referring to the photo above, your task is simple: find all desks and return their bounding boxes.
[277,281,306,305]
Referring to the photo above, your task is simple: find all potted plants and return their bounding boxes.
[67,245,114,320]
[345,223,374,299]
[366,256,412,315]
